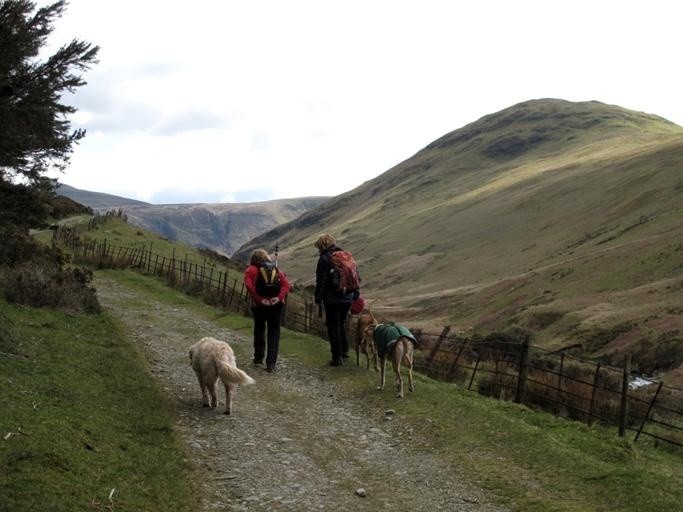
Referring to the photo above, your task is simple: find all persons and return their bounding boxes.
[313,231,362,365]
[242,249,289,373]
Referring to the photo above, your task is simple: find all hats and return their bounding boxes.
[351,298,365,314]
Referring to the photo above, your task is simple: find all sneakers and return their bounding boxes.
[342,354,349,359]
[254,359,263,367]
[330,359,344,366]
[267,364,275,372]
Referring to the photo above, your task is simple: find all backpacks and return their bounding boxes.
[326,251,361,294]
[255,260,282,298]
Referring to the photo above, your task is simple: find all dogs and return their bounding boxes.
[369,323,415,398]
[355,305,379,372]
[189,336,257,415]
